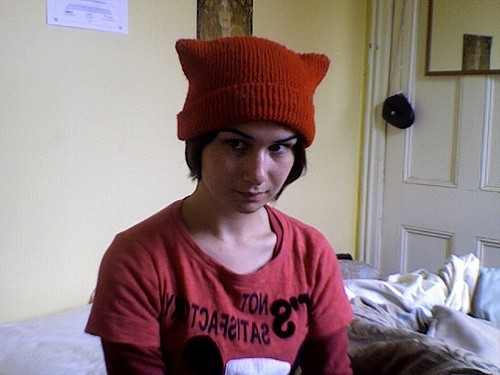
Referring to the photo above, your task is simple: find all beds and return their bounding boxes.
[0,253,500,375]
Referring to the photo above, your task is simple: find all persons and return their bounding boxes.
[83,36,354,375]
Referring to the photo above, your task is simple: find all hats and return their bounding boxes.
[174,36,333,148]
